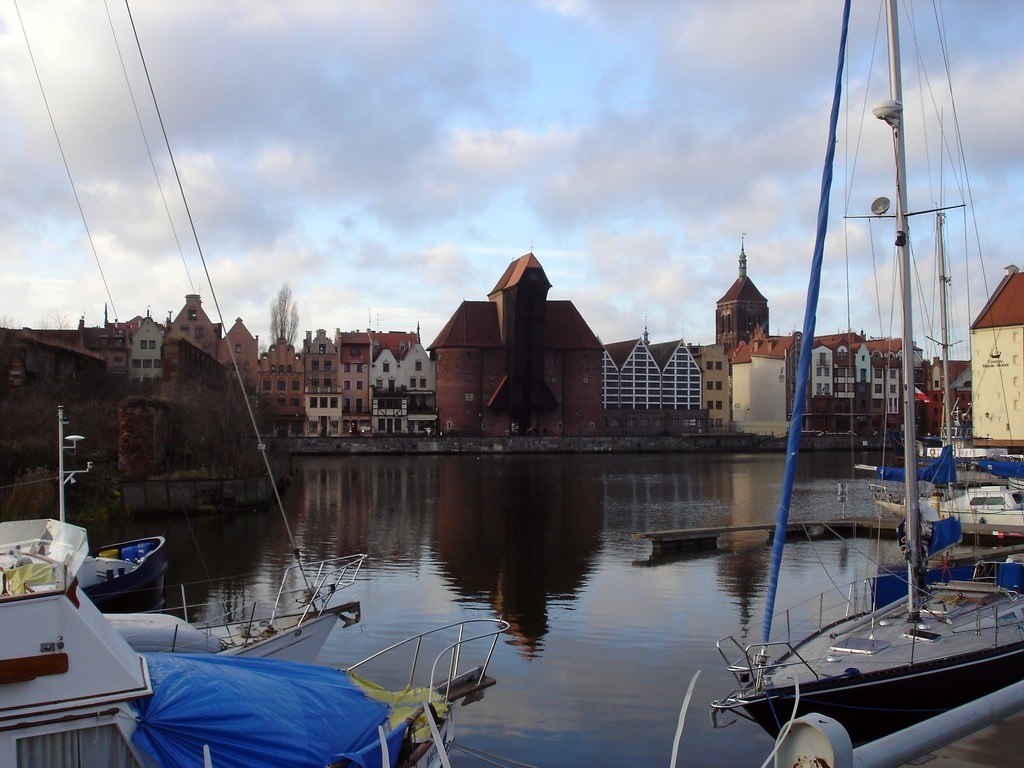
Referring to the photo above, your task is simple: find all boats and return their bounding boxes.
[869,480,1023,539]
[2,539,512,768]
[0,407,171,613]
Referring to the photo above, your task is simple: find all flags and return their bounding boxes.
[915,387,933,404]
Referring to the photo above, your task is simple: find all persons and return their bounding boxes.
[979,517,985,524]
[699,428,702,434]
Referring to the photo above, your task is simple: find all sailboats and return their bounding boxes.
[700,5,1024,751]
[855,211,1023,489]
[0,4,370,670]
